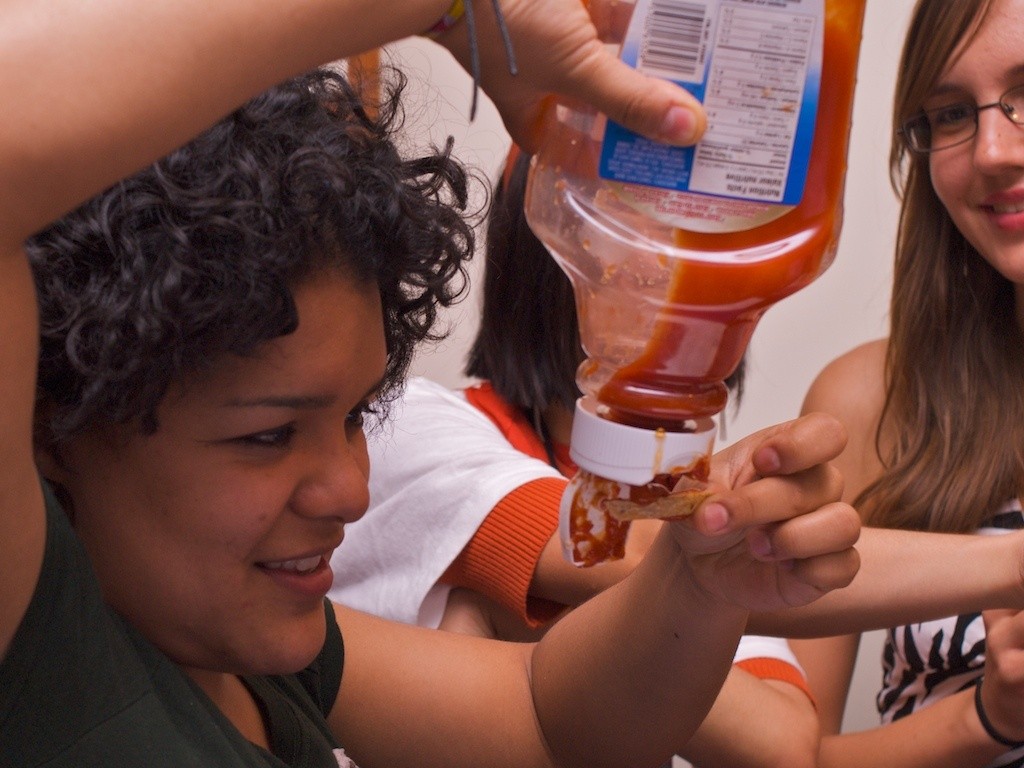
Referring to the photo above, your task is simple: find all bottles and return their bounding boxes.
[522,0,868,568]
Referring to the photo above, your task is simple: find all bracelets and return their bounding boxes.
[975,674,1023,749]
[419,0,518,123]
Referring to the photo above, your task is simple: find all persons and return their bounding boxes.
[319,133,1024,768]
[0,0,863,768]
[782,0,1024,767]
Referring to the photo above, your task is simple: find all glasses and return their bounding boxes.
[897,85,1024,153]
